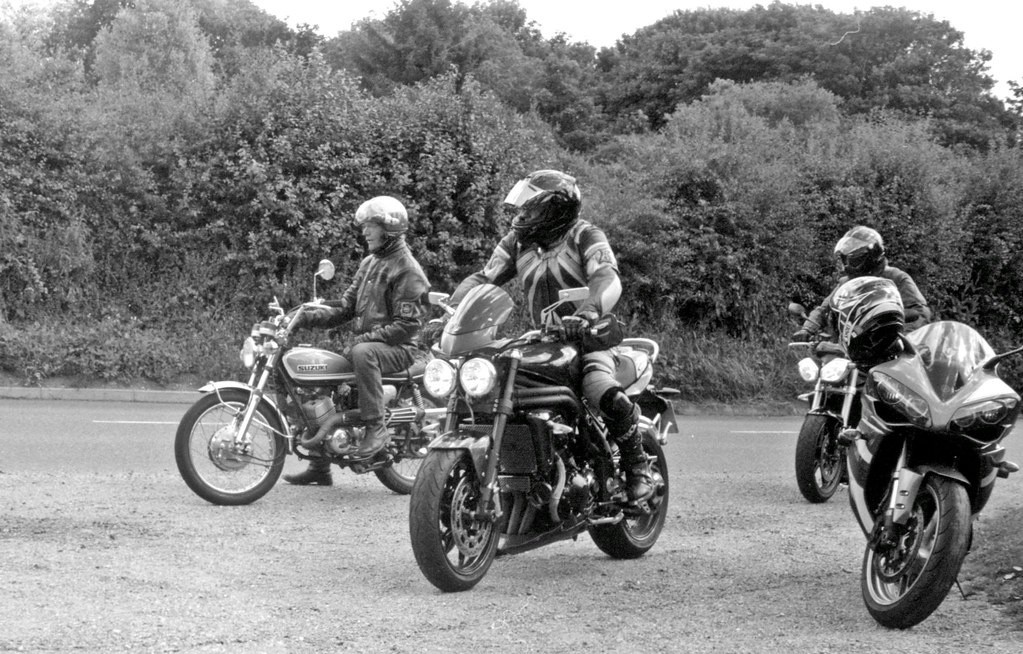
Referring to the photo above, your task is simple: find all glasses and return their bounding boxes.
[842,253,868,267]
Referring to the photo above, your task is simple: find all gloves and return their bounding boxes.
[559,311,600,345]
[423,319,444,345]
[792,330,811,342]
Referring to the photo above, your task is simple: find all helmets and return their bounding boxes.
[829,276,905,360]
[839,226,885,276]
[356,195,408,254]
[512,170,581,247]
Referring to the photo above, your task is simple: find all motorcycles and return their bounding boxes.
[405,280,683,594]
[174,259,457,508]
[786,302,866,504]
[836,317,1023,629]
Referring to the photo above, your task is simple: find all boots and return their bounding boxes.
[603,403,654,506]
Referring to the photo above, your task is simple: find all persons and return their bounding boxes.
[419,170,656,504]
[268,197,431,485]
[793,225,930,482]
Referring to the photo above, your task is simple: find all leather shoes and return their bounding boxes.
[283,465,332,486]
[357,417,391,456]
